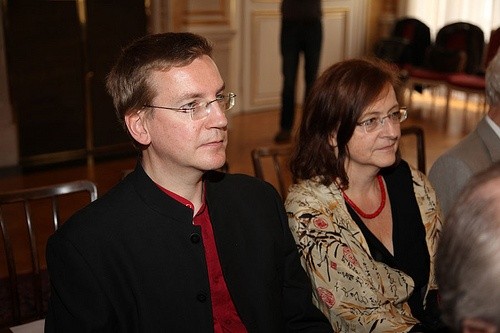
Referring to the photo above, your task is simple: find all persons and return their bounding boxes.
[43,34,331,333]
[273,0,324,143]
[284,56,445,333]
[434,163,500,333]
[428,51,500,209]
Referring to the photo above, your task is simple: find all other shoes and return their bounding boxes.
[273,133,292,142]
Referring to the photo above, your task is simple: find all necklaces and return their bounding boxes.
[334,173,385,218]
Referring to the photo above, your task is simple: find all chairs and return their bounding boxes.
[250,126,426,202]
[0,179,98,333]
[366,18,500,113]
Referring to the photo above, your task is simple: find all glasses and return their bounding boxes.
[142,92,237,120]
[356,104,408,133]
